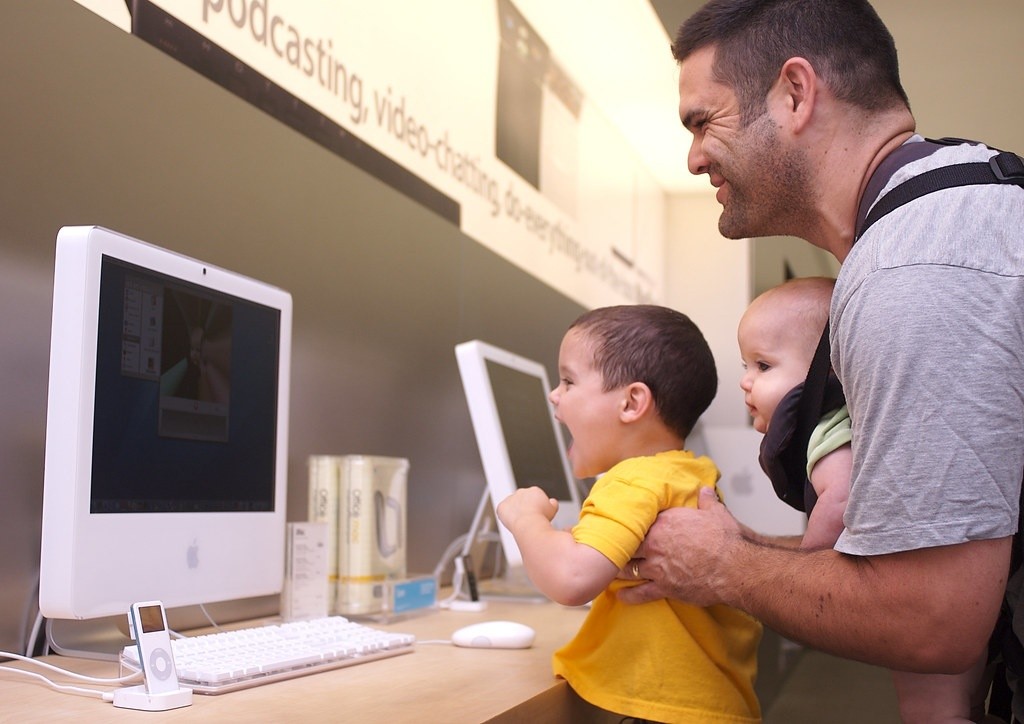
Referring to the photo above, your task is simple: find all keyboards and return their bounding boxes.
[121,615,415,694]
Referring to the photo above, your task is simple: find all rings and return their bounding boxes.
[631,560,642,579]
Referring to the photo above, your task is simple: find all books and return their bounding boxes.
[308,454,338,610]
[339,453,408,613]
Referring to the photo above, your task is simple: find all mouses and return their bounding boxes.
[451,621,535,649]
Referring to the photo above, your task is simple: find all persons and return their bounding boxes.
[615,0,1022,724]
[737,275,987,724]
[189,322,205,367]
[494,303,762,723]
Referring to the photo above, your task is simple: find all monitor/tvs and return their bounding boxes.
[38,226,296,618]
[455,339,585,568]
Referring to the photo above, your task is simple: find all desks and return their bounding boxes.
[0,574,594,724]
